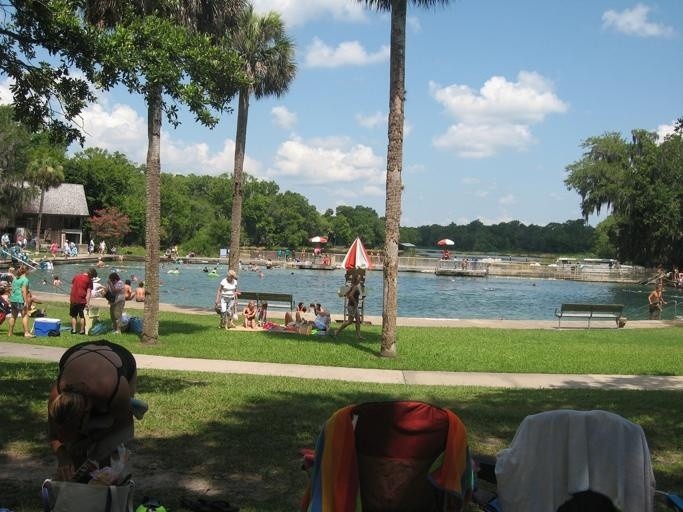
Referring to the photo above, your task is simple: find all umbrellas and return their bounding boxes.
[340,235,371,272]
[307,235,327,244]
[436,238,455,246]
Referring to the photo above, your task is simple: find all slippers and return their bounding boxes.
[182,498,212,512]
[196,499,241,512]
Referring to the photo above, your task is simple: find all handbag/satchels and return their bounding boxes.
[88,312,144,336]
[38,477,137,512]
[232,313,239,321]
[102,288,116,304]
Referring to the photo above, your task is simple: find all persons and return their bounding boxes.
[444,249,449,259]
[0,229,145,338]
[453,255,457,268]
[334,277,368,340]
[647,263,683,319]
[461,259,465,270]
[162,244,331,336]
[45,338,137,482]
[464,258,469,270]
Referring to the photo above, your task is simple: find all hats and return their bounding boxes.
[92,276,102,283]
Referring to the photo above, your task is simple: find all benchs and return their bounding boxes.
[236,291,295,316]
[554,303,624,330]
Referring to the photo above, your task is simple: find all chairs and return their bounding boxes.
[653,489,683,512]
[474,409,656,512]
[300,400,472,512]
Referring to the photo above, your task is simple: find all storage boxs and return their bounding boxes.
[33,317,62,337]
